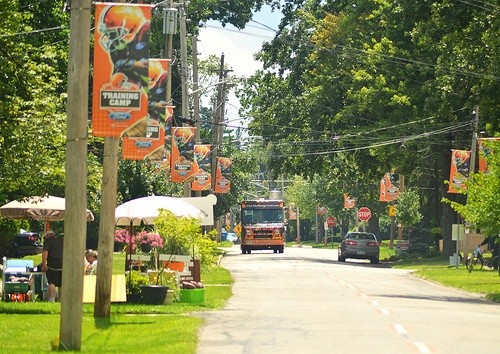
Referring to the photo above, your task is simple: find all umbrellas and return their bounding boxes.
[0,195,94,235]
[114,193,208,284]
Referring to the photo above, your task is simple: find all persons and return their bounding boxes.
[194,146,212,173]
[85,250,96,276]
[219,158,230,181]
[98,5,151,97]
[148,61,165,126]
[41,232,63,301]
[174,127,195,163]
[491,243,500,270]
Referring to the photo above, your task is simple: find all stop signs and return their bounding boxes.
[357,207,371,221]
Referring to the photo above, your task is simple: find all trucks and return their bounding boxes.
[238,200,289,254]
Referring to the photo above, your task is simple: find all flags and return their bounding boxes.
[478,139,495,174]
[318,205,326,214]
[449,151,471,192]
[289,206,297,219]
[344,194,354,208]
[215,158,231,193]
[91,4,151,132]
[171,128,195,180]
[385,174,400,200]
[380,178,386,200]
[192,146,212,189]
[121,61,168,156]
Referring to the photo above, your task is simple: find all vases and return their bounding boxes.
[139,285,169,304]
[140,244,151,252]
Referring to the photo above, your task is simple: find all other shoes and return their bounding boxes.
[48,296,55,303]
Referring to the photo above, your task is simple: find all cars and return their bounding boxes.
[226,233,238,244]
[337,231,380,263]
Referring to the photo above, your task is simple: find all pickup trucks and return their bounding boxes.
[13,232,39,247]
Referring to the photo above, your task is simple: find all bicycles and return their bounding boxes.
[466,244,499,271]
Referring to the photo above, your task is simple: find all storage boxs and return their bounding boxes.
[180,288,205,303]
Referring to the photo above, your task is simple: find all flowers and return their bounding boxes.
[114,229,163,248]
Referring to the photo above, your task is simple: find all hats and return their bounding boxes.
[43,230,53,239]
